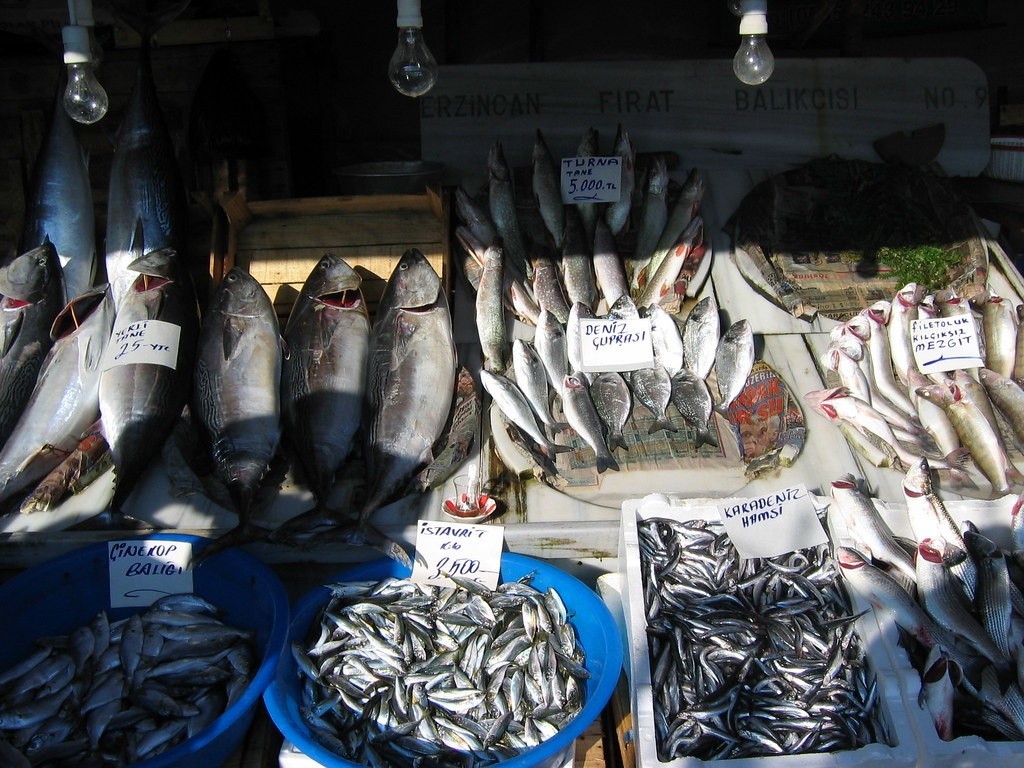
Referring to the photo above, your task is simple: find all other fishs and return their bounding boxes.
[0,32,1024,768]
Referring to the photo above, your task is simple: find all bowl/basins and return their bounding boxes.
[982,134,1024,183]
[331,160,446,196]
[0,533,291,768]
[441,495,497,524]
[262,551,624,768]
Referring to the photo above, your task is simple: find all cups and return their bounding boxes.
[453,474,480,518]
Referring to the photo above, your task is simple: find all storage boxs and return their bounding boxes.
[222,191,454,315]
[0,241,1024,768]
